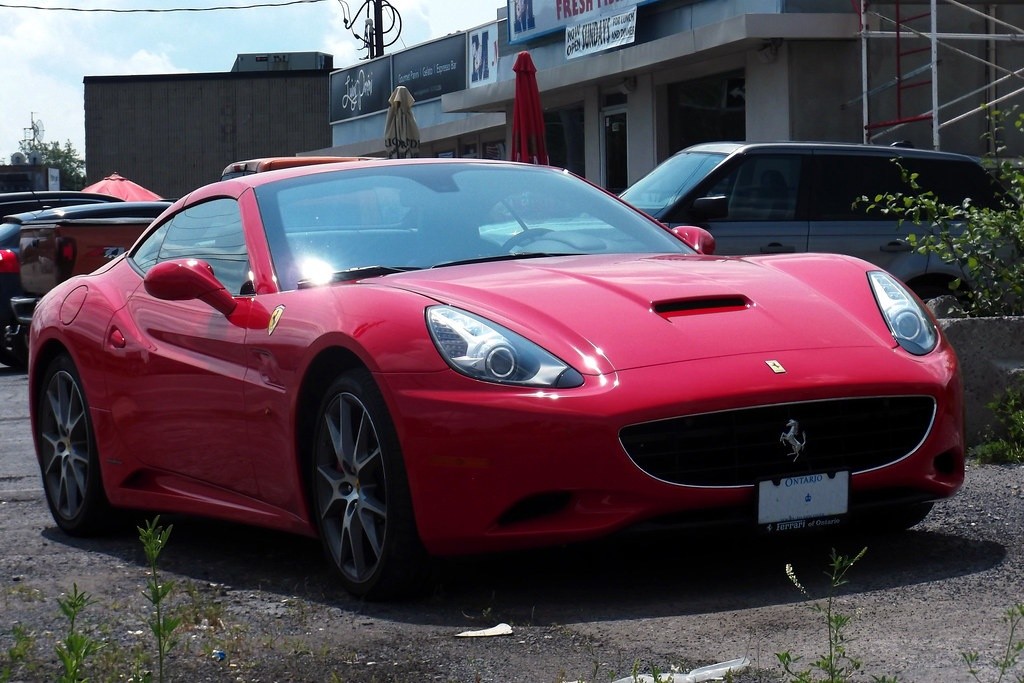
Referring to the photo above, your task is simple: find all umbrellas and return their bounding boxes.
[513,51,550,165]
[82,172,165,202]
[384,86,420,159]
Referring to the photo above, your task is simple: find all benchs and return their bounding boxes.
[285,230,413,271]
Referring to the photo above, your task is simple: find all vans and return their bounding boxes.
[219,159,385,179]
[476,138,1020,321]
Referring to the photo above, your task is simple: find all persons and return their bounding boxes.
[471,35,489,82]
[514,0,535,32]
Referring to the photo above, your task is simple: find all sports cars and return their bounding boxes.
[23,165,970,601]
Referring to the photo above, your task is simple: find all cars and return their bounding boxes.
[1,190,221,370]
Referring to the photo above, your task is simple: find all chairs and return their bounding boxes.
[411,194,503,268]
